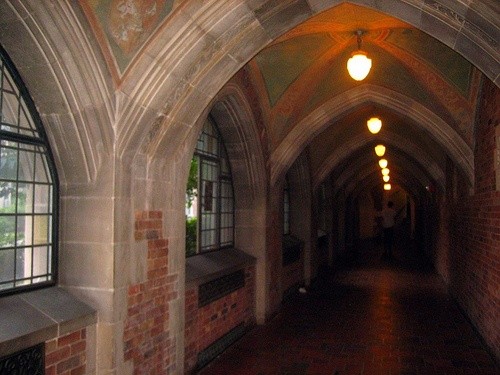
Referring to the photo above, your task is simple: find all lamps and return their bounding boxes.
[343,30,373,83]
